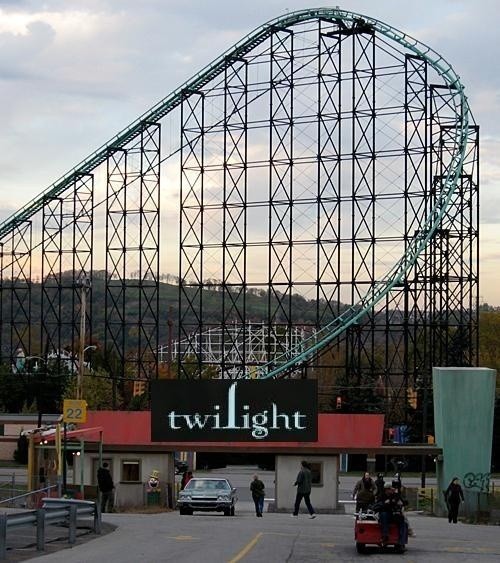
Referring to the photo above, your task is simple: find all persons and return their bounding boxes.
[351,470,409,549]
[291,459,317,517]
[96,462,115,513]
[180,470,194,490]
[250,474,266,517]
[445,475,466,522]
[216,482,226,489]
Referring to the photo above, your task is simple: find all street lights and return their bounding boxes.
[76,275,98,399]
[415,374,433,496]
[25,355,48,427]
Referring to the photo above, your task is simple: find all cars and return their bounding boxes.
[174,455,189,475]
[21,423,75,438]
[175,477,238,517]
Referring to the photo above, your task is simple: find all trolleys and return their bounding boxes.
[354,509,409,553]
